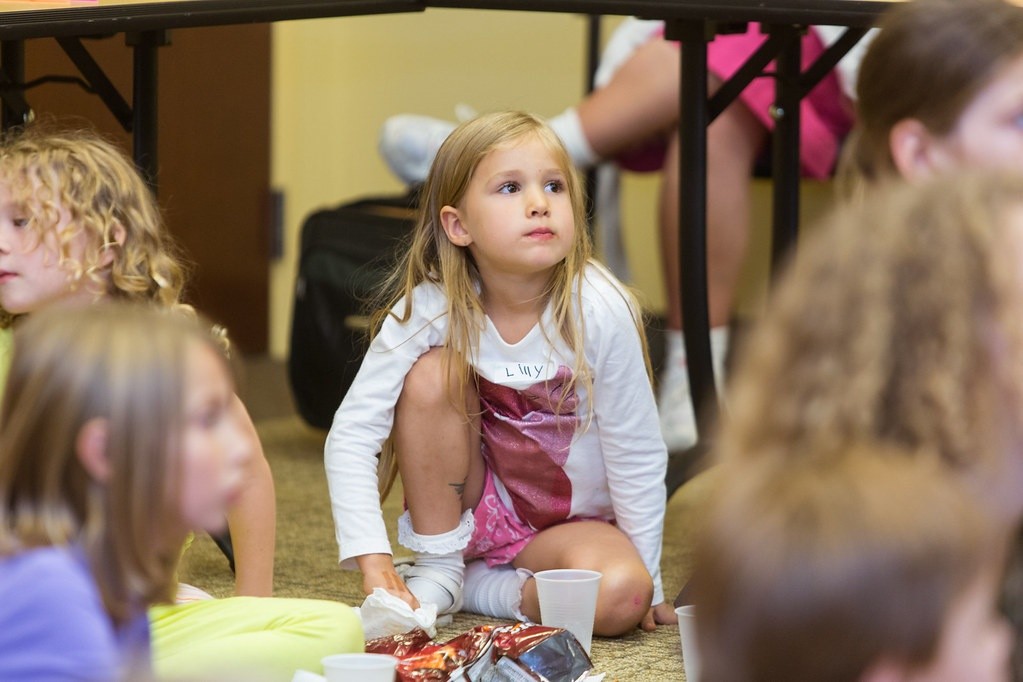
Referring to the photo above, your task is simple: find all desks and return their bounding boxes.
[0,0,892,445]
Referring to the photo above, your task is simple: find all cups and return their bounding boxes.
[674,605,708,682]
[535,569,603,658]
[321,652,399,682]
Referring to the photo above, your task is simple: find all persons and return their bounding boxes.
[0,134,365,682]
[380,15,882,453]
[690,0,1023,681]
[322,109,677,636]
[0,296,255,682]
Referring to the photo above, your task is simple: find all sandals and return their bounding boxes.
[397,567,464,615]
[392,557,415,577]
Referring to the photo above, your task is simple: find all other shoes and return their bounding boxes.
[378,100,481,185]
